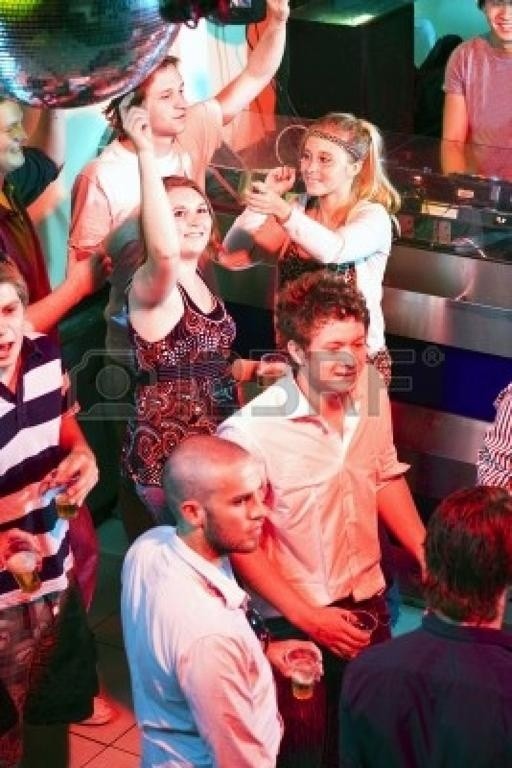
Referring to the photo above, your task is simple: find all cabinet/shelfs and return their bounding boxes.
[96,105,511,520]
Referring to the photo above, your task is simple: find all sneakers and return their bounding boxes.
[74,697,114,726]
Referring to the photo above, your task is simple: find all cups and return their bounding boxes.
[346,610,378,635]
[53,469,79,517]
[68,239,102,262]
[284,649,319,700]
[4,543,42,593]
[259,353,288,390]
